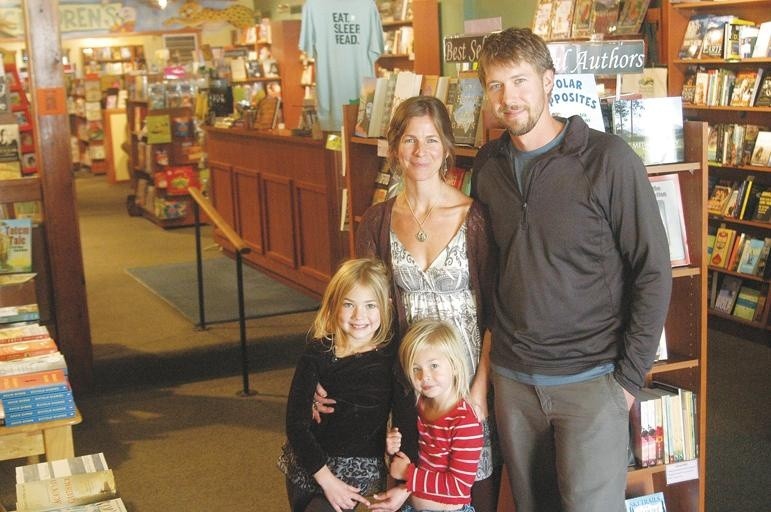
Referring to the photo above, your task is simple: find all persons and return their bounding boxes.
[467,29,677,511]
[385,315,486,511]
[275,257,421,511]
[312,94,503,511]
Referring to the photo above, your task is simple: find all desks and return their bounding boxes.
[0,400,82,467]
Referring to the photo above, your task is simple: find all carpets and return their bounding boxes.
[123,254,322,326]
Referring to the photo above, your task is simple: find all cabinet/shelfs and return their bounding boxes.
[658,0,771,342]
[489,119,712,512]
[376,18,414,64]
[342,104,505,265]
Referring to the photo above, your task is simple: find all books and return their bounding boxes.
[351,1,692,269]
[624,377,699,470]
[1,323,133,511]
[679,13,771,320]
[0,37,45,325]
[656,324,669,364]
[63,46,283,223]
[709,234,771,330]
[627,491,670,512]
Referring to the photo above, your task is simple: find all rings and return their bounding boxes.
[312,397,320,409]
